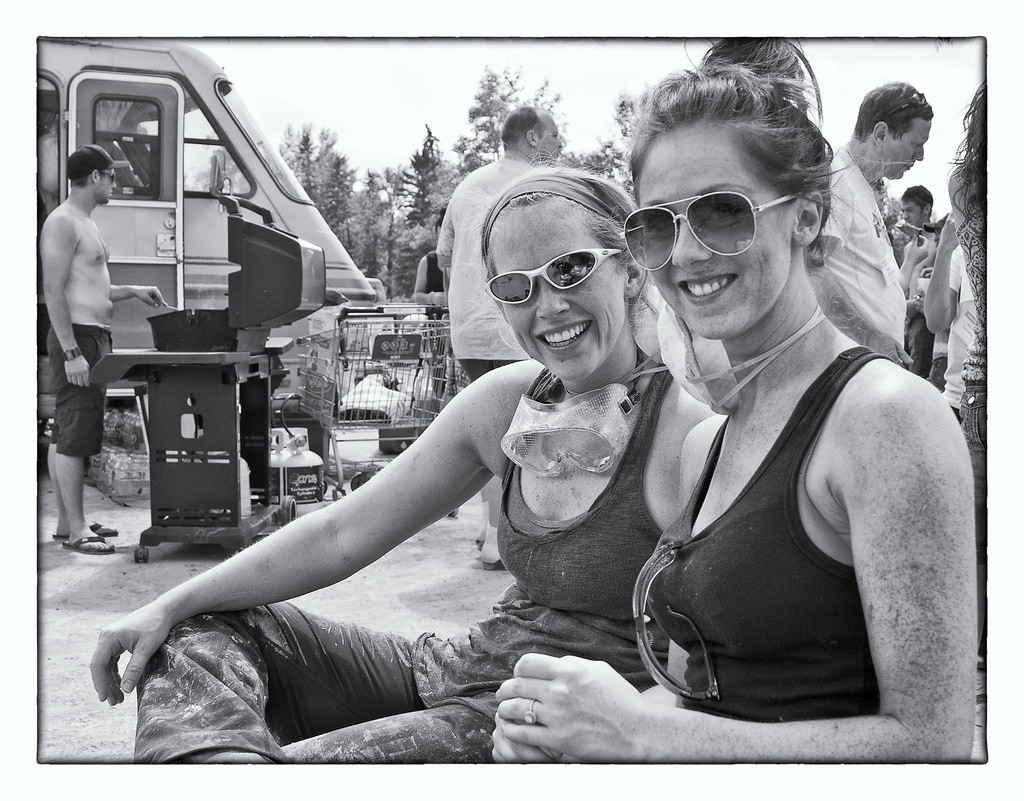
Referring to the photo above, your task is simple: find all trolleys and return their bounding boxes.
[296,303,450,502]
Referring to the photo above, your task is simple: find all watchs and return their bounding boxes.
[63,347,84,361]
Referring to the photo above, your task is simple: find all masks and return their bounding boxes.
[657,304,740,416]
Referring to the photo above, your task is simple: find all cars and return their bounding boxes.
[338,278,388,315]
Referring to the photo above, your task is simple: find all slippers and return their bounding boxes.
[62,536,115,555]
[53,521,118,538]
[482,559,507,571]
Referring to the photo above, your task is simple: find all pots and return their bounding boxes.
[146,307,238,353]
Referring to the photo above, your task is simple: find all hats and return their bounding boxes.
[67,145,114,180]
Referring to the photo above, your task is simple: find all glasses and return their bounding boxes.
[99,172,114,183]
[486,249,624,304]
[619,193,801,271]
[500,345,645,479]
[633,540,720,701]
[880,92,927,121]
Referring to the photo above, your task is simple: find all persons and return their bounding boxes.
[806,76,986,668]
[434,105,564,572]
[89,164,716,765]
[486,36,977,764]
[39,145,167,556]
[412,206,456,401]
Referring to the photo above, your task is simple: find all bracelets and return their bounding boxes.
[426,291,434,304]
[914,294,921,313]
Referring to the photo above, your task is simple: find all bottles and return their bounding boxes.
[86,442,150,496]
[103,408,144,453]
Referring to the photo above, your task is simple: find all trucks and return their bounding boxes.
[38,38,390,438]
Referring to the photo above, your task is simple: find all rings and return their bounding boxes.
[523,700,537,724]
